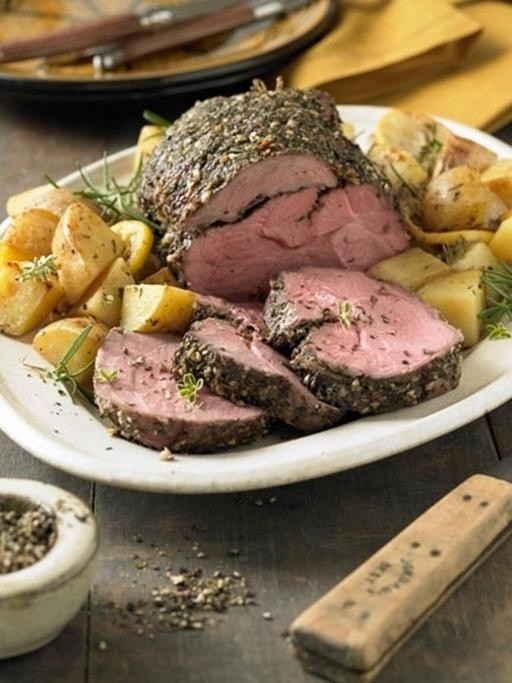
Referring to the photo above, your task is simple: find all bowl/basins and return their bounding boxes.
[0,477,99,660]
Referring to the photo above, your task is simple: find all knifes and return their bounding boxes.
[94,1,306,74]
[2,3,222,61]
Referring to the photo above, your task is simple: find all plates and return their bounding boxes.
[2,1,342,121]
[2,100,512,495]
